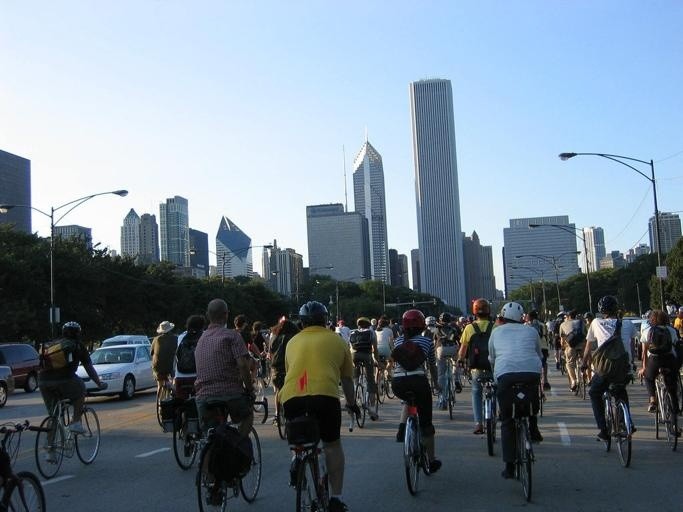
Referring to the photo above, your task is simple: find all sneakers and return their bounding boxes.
[396,431,405,442]
[596,432,610,443]
[429,460,442,474]
[473,424,483,434]
[500,469,514,479]
[45,453,58,464]
[648,403,657,413]
[369,407,378,420]
[455,380,462,393]
[288,459,307,490]
[327,498,352,512]
[438,400,448,410]
[531,428,543,443]
[70,423,88,433]
[543,359,578,393]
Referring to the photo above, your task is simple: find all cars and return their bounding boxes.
[0,365,16,408]
[72,343,160,399]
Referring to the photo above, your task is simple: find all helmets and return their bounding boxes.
[598,296,619,314]
[62,321,83,336]
[439,312,452,323]
[357,317,371,325]
[472,298,490,315]
[425,316,436,325]
[501,302,524,323]
[61,321,82,333]
[299,301,329,318]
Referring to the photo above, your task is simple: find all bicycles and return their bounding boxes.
[30,381,108,480]
[0,419,51,512]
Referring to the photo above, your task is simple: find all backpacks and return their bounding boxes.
[550,319,563,334]
[468,322,494,370]
[351,331,372,352]
[647,326,673,354]
[437,325,456,345]
[178,331,203,374]
[39,338,76,373]
[393,339,426,371]
[527,322,544,339]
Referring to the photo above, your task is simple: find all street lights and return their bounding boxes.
[267,264,335,313]
[525,222,595,316]
[0,188,133,345]
[355,271,405,322]
[312,274,369,328]
[186,242,275,303]
[504,246,583,316]
[556,150,668,314]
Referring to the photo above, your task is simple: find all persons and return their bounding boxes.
[36,320,108,463]
[148,295,683,511]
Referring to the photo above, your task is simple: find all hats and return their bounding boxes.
[371,318,377,325]
[557,311,566,317]
[337,320,345,325]
[402,309,426,329]
[156,321,175,333]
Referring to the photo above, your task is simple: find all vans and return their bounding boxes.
[99,332,152,346]
[0,342,41,391]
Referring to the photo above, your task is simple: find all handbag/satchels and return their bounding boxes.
[208,423,254,483]
[566,327,585,346]
[589,319,631,382]
[254,335,263,353]
